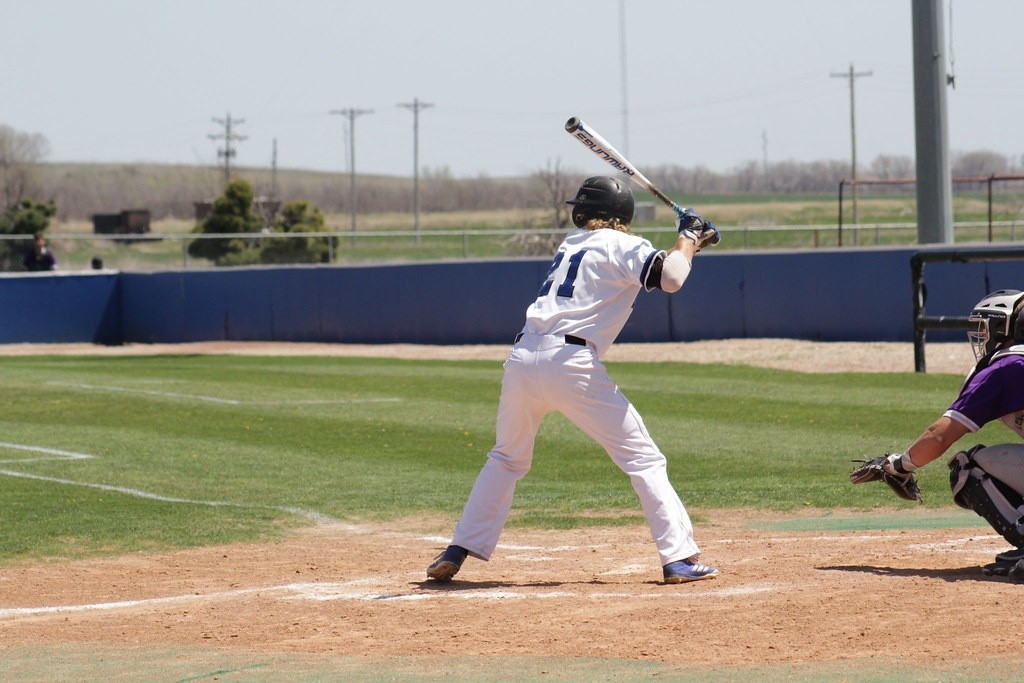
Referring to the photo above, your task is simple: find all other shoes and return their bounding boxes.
[995,549,1024,564]
[981,559,1024,576]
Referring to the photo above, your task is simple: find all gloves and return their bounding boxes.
[694,219,721,253]
[674,205,705,245]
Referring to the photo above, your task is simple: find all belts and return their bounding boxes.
[515,333,586,347]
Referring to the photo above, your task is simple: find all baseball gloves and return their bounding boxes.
[847,452,925,505]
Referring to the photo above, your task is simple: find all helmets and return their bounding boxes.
[566,175,634,229]
[967,290,1024,364]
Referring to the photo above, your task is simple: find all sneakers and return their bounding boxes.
[663,558,719,583]
[426,543,468,580]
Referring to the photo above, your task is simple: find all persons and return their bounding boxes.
[24,233,57,272]
[92,257,103,269]
[850,290,1024,577]
[426,176,720,582]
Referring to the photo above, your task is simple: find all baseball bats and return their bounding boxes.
[563,116,722,247]
